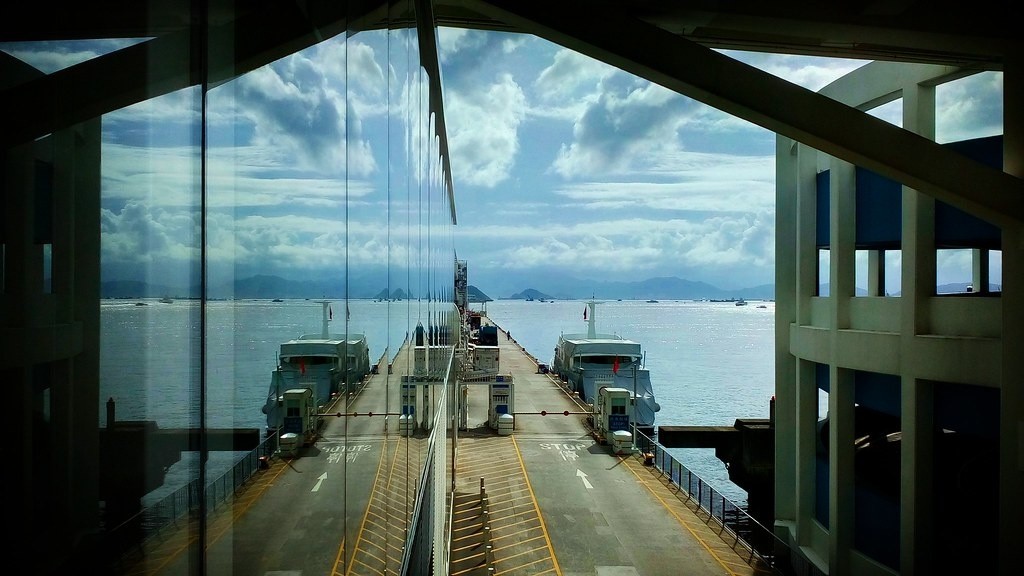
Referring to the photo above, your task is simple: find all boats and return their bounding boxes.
[263,300,370,432]
[549,290,660,446]
[646,300,658,303]
[735,298,747,306]
[271,298,283,302]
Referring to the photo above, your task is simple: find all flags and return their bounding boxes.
[613,356,620,373]
[329,307,332,319]
[300,358,305,377]
[583,307,587,319]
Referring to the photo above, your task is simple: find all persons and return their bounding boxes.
[507,331,510,340]
